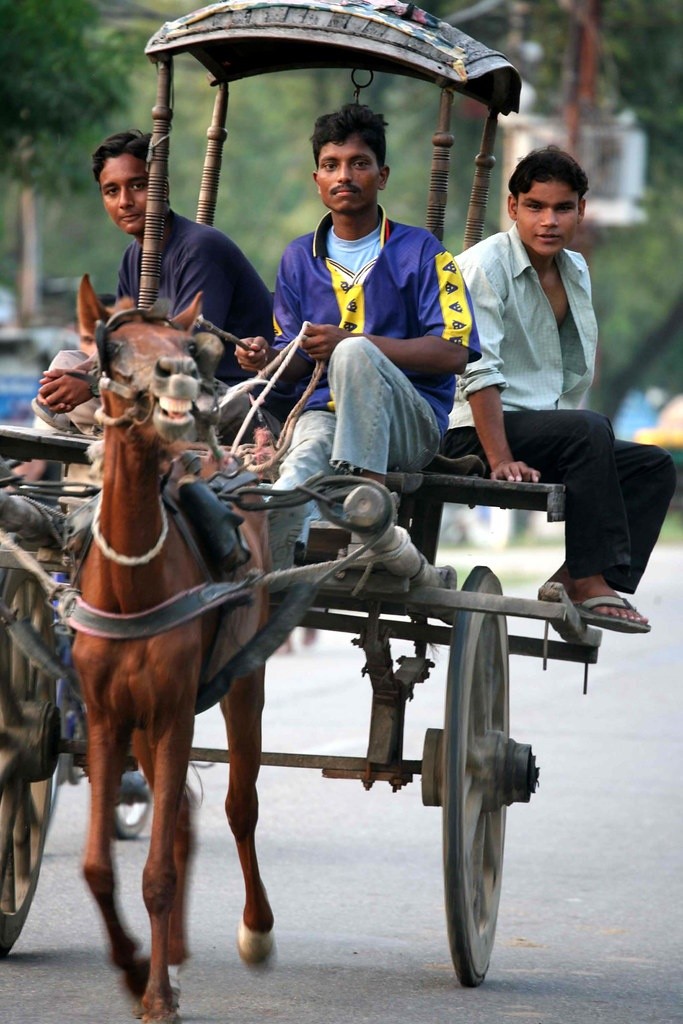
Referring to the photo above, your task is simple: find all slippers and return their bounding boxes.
[574,596,652,635]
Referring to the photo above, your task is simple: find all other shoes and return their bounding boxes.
[31,398,82,434]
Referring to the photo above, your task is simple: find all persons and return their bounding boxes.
[36,130,284,443]
[442,148,677,632]
[228,100,484,594]
[0,294,118,763]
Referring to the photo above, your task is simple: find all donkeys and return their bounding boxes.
[61,271,273,1024]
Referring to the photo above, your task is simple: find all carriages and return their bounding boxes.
[0,0,598,1024]
